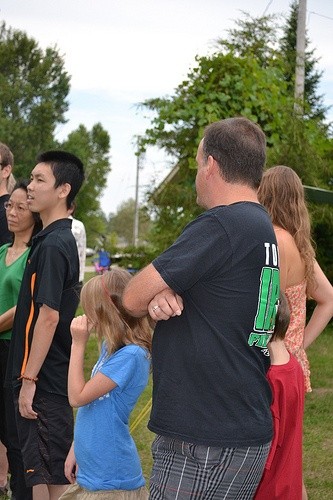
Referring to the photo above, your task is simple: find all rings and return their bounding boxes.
[153,306,159,312]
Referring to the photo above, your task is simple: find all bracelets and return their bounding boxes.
[17,375,38,381]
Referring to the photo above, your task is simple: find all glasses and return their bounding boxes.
[3,202,29,213]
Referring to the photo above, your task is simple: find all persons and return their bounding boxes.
[254,291,305,500]
[0,181,43,500]
[58,251,153,500]
[0,143,17,500]
[4,150,85,500]
[258,166,333,500]
[122,118,280,500]
[68,201,86,304]
